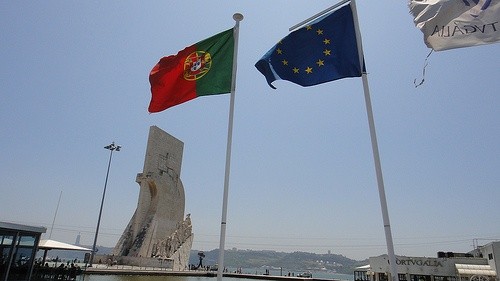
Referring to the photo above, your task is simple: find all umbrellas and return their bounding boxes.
[0,237,95,266]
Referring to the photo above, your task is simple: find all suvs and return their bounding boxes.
[297,272,312,279]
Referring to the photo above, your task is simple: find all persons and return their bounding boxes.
[0,255,77,281]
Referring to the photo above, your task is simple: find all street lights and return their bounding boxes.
[89,143,121,268]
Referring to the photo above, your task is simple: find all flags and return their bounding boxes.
[147,28,235,113]
[254,5,367,86]
[408,0,500,53]
